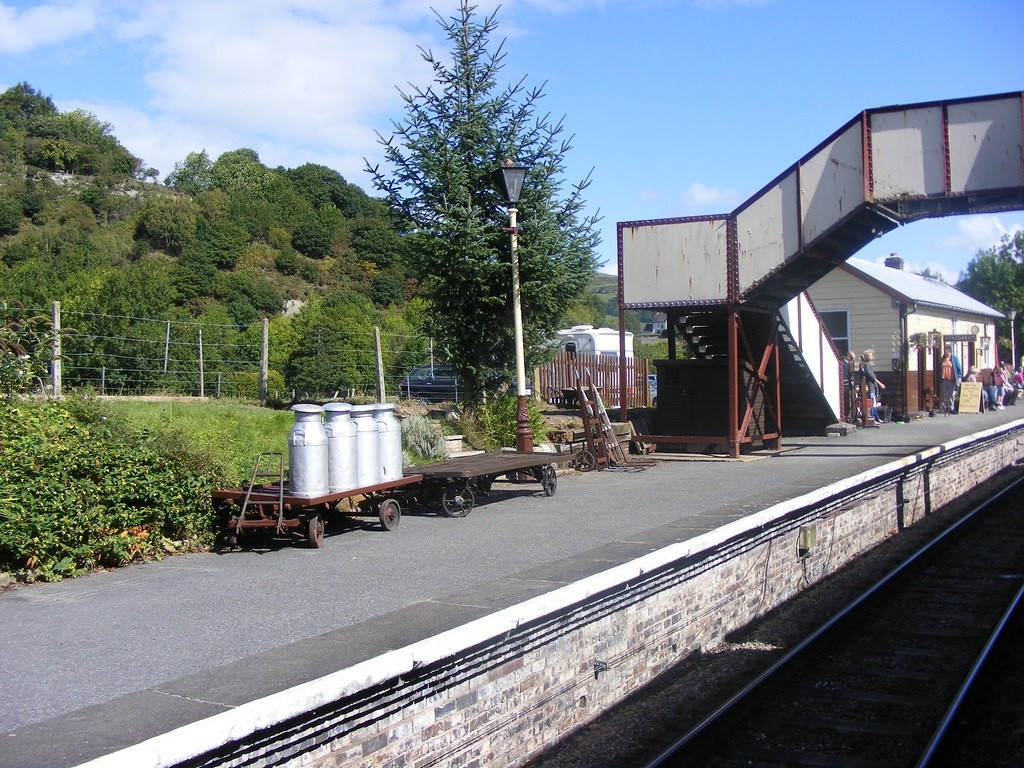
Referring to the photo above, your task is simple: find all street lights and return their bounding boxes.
[1007,307,1017,371]
[491,162,539,482]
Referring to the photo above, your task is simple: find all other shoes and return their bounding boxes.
[879,419,884,423]
[951,410,957,414]
[990,407,996,411]
[875,421,880,424]
[993,401,999,406]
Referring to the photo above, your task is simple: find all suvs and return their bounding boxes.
[398,362,535,406]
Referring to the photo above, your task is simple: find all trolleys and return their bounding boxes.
[572,366,606,471]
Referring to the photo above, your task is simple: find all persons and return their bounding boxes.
[965,361,1024,411]
[938,345,962,414]
[843,350,885,423]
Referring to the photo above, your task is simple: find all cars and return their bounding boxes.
[634,374,658,408]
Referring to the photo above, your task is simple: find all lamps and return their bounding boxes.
[977,335,990,357]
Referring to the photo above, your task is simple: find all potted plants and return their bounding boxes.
[911,332,930,353]
